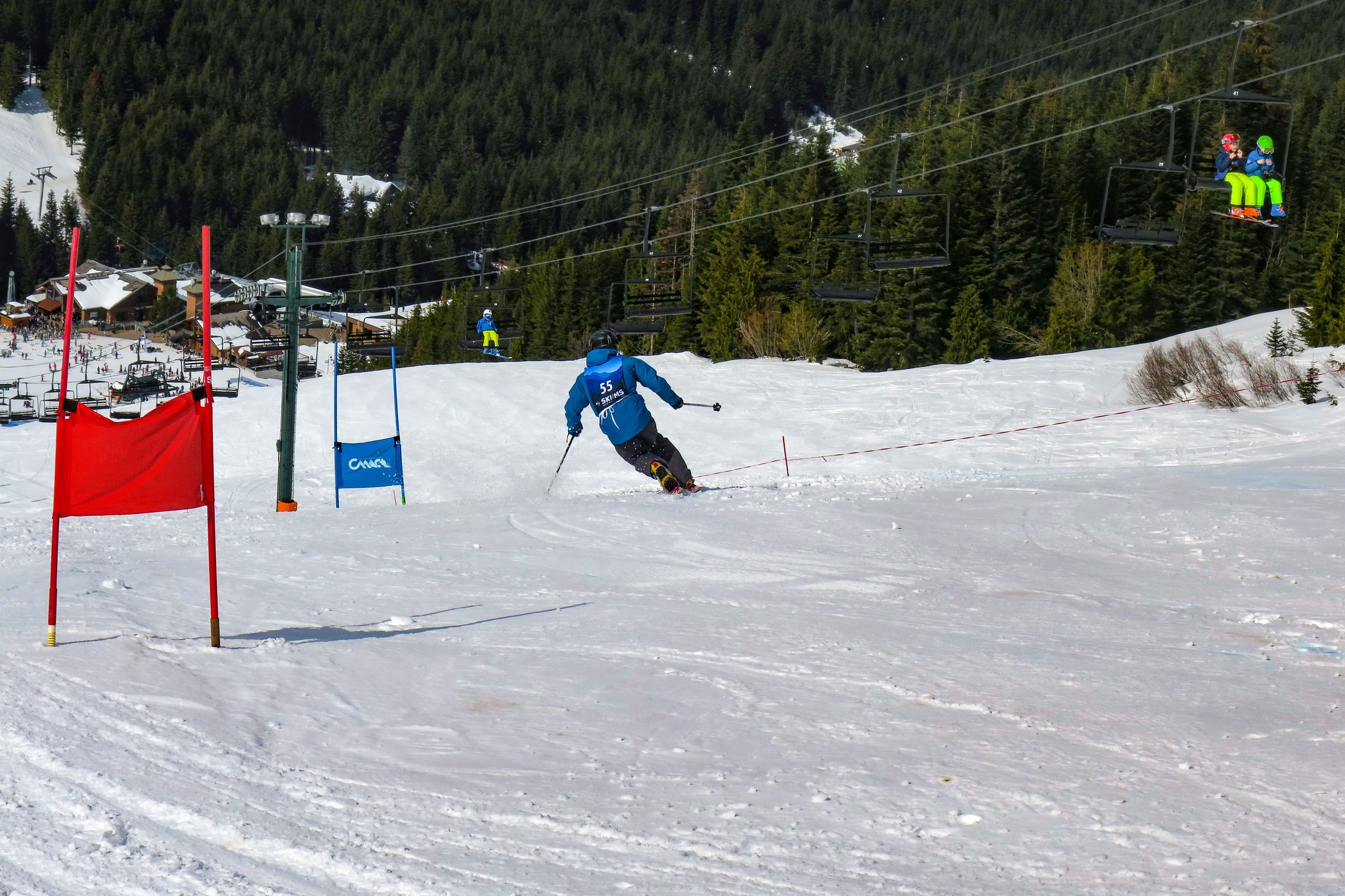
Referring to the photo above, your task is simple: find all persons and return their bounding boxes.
[312,311,315,318]
[53,363,56,370]
[304,314,306,318]
[41,374,44,381]
[269,329,271,334]
[8,314,65,360]
[1245,136,1285,218]
[227,380,232,387]
[441,296,443,303]
[1214,133,1259,216]
[281,328,284,335]
[189,372,203,389]
[564,330,700,494]
[332,322,346,333]
[69,329,107,373]
[477,309,499,355]
[97,355,179,380]
[173,385,184,395]
[49,363,51,371]
[26,401,30,408]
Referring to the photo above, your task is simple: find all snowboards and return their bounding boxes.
[480,348,512,359]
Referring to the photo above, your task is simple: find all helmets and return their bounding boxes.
[483,310,492,317]
[589,329,619,350]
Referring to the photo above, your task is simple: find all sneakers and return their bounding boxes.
[493,349,499,355]
[651,462,680,492]
[483,347,490,354]
[683,478,702,493]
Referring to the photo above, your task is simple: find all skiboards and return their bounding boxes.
[1209,208,1272,223]
[659,486,719,496]
[1246,215,1279,227]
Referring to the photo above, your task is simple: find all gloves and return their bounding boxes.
[479,332,483,335]
[568,425,583,437]
[671,398,684,410]
